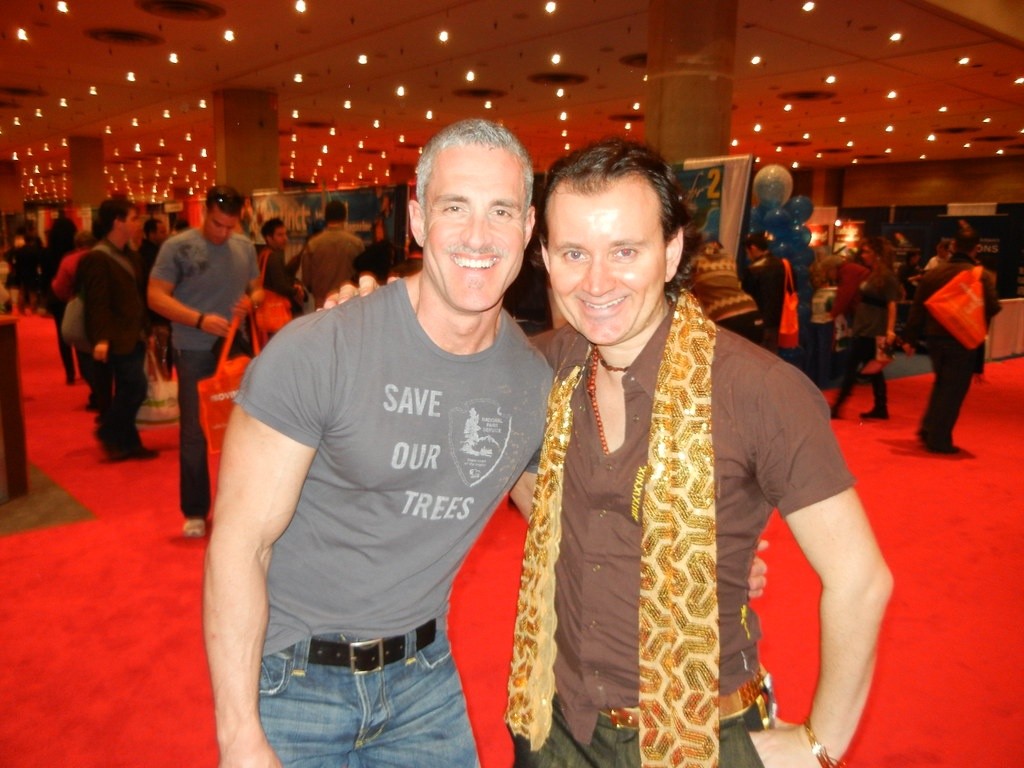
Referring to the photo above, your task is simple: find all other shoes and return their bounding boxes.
[861,406,888,420]
[917,430,959,455]
[96,426,159,461]
[831,409,839,419]
[183,517,206,537]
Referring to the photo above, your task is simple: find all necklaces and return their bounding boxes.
[587,345,629,456]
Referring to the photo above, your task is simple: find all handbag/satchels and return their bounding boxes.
[778,258,799,348]
[198,298,262,452]
[249,247,291,335]
[61,295,92,353]
[135,350,180,430]
[924,266,987,350]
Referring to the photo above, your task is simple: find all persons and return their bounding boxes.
[202,118,768,768]
[0,194,192,462]
[903,227,1002,452]
[682,237,758,339]
[740,231,785,356]
[806,234,957,418]
[286,198,423,309]
[316,132,895,768]
[148,180,264,539]
[256,218,305,318]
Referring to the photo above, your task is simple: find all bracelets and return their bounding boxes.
[804,718,846,767]
[196,315,204,329]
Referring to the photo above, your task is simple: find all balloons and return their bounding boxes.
[746,163,814,309]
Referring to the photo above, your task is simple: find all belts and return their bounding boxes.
[596,670,764,730]
[307,619,436,674]
